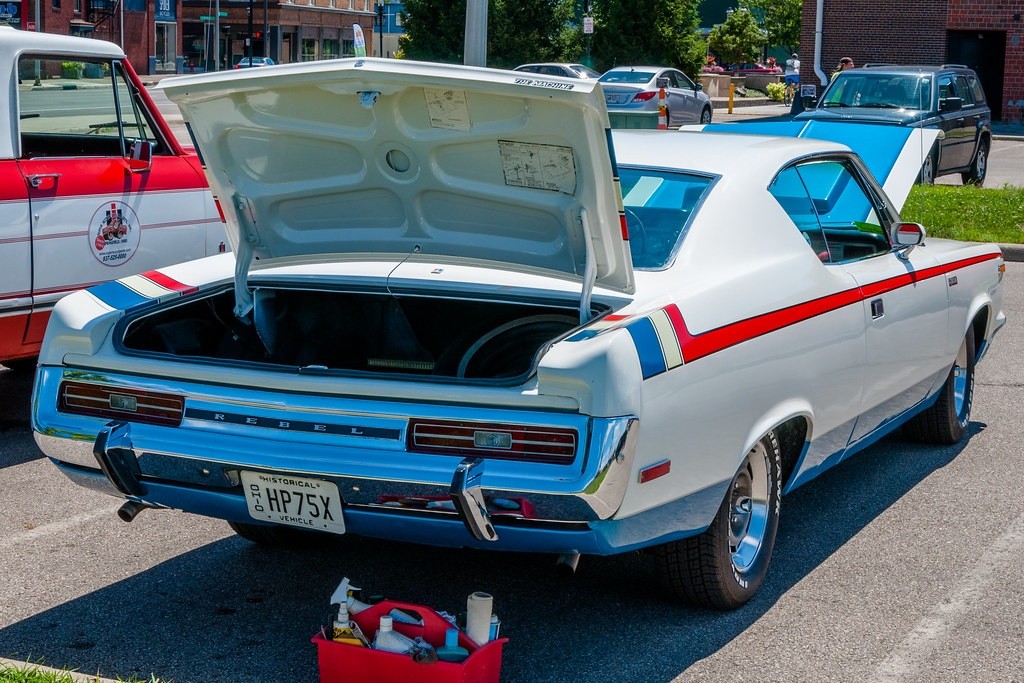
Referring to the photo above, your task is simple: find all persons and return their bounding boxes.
[828,57,860,106]
[943,83,962,110]
[785,53,800,106]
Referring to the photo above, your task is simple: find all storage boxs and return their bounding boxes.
[310,600,509,683]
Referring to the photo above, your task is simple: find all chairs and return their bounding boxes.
[880,85,908,106]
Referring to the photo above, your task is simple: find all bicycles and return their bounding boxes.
[783,79,794,107]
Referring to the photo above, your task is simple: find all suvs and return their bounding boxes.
[812,63,994,186]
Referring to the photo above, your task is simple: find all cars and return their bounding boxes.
[233,57,275,69]
[513,61,601,80]
[30,56,1010,616]
[593,65,714,130]
[0,23,232,366]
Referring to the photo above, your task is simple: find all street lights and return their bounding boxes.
[377,0,385,58]
